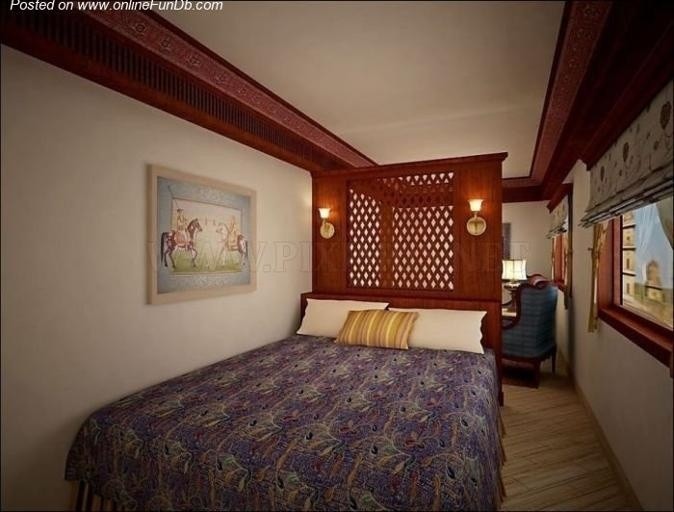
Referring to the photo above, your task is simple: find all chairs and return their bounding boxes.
[499,282,558,389]
[500,274,546,322]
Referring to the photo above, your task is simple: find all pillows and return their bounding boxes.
[386,305,488,358]
[335,309,418,352]
[294,296,390,340]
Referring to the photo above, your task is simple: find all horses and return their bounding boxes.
[160,218,204,269]
[215,222,249,272]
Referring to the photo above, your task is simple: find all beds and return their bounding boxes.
[61,290,505,510]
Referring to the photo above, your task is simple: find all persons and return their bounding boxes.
[173,209,190,250]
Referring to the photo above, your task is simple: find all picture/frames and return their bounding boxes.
[144,162,258,305]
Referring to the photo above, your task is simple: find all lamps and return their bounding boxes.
[317,206,335,238]
[463,199,488,238]
[502,259,528,285]
